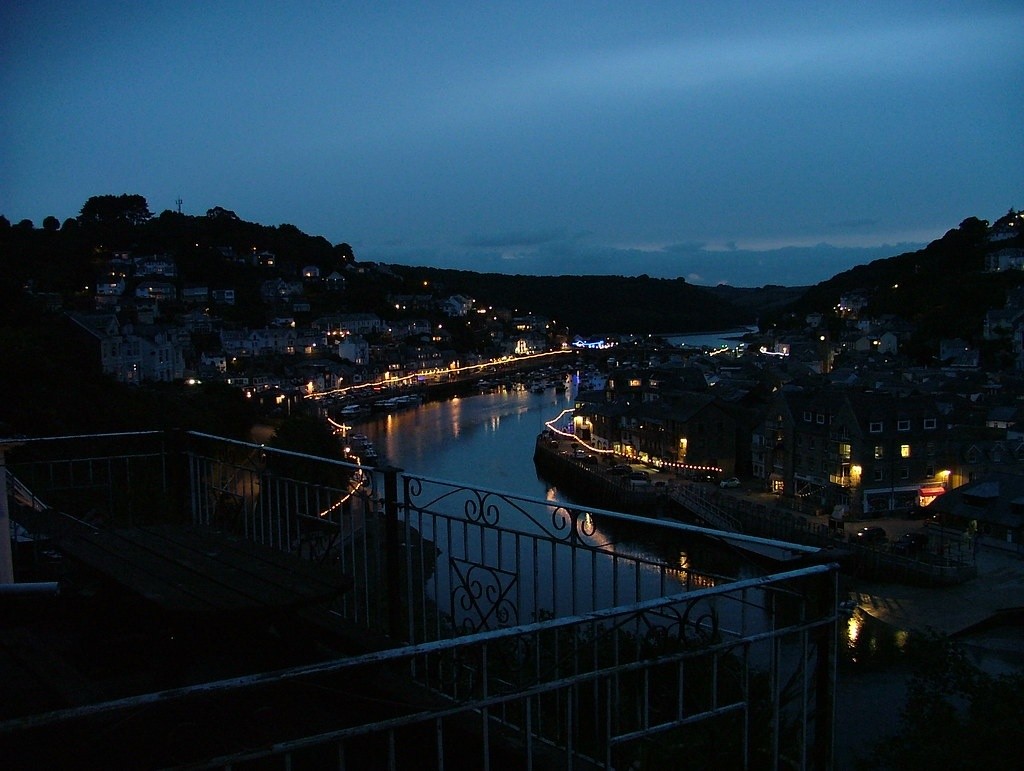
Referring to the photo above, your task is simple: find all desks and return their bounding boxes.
[49,523,343,673]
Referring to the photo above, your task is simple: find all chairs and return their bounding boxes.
[296,512,342,565]
[210,486,244,531]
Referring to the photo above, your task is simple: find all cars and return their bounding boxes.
[897,531,928,556]
[568,449,598,467]
[606,464,633,475]
[719,477,742,488]
[621,469,649,482]
[691,470,721,483]
[857,525,885,544]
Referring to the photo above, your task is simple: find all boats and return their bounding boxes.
[341,403,363,421]
[374,393,423,416]
